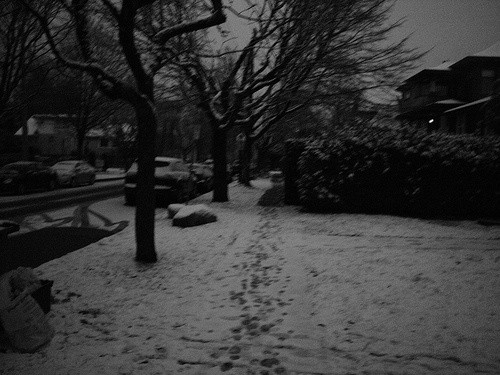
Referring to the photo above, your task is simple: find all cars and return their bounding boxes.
[51,158,99,190]
[129,156,236,184]
[1,161,61,195]
